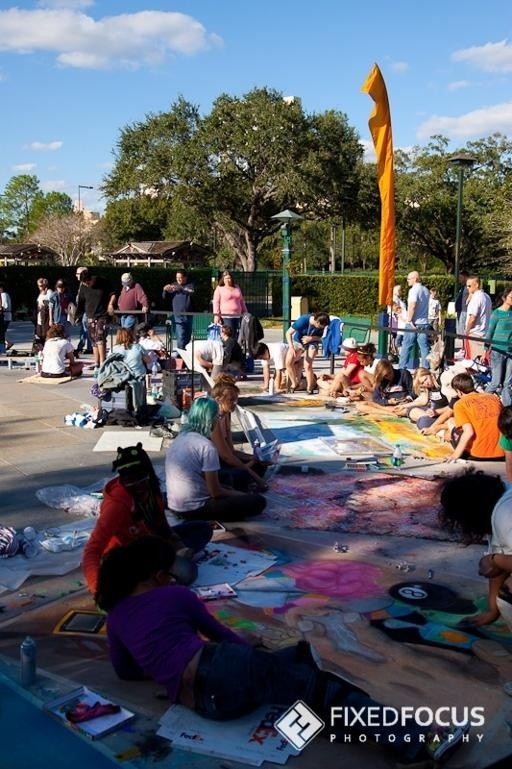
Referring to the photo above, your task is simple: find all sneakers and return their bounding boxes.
[425,719,470,764]
[85,363,99,369]
[150,426,178,439]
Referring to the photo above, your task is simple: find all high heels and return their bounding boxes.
[306,379,317,396]
[288,383,299,394]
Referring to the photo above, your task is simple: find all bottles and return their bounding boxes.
[151,361,158,379]
[392,444,403,470]
[19,636,37,688]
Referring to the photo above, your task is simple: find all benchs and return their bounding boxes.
[338,315,373,346]
[165,313,215,355]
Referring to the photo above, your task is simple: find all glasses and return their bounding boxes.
[75,272,80,274]
[123,474,150,488]
[467,285,471,287]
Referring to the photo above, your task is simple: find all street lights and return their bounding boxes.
[444,154,477,366]
[272,209,310,345]
[76,183,95,267]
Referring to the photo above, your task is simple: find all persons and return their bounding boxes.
[118,271,150,329]
[464,275,493,360]
[438,467,511,634]
[396,269,434,370]
[444,372,506,462]
[211,269,249,337]
[33,278,54,348]
[47,279,70,328]
[106,536,473,768]
[320,337,365,399]
[79,444,214,613]
[392,363,448,429]
[341,341,385,399]
[182,337,230,385]
[454,269,471,359]
[425,286,442,352]
[162,396,269,522]
[479,285,511,404]
[1,287,16,353]
[202,382,269,495]
[216,323,247,381]
[106,326,153,389]
[72,269,119,372]
[371,356,418,407]
[134,321,168,370]
[250,341,319,393]
[284,311,331,394]
[161,268,196,352]
[497,404,512,485]
[69,266,94,355]
[386,283,406,357]
[39,324,85,378]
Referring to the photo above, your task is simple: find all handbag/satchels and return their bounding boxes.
[207,324,222,340]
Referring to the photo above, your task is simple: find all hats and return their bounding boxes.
[111,443,145,471]
[122,273,133,285]
[342,337,358,349]
[54,279,69,288]
